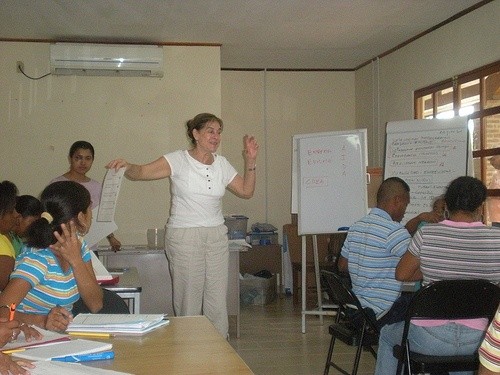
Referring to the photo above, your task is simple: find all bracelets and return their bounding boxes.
[245,168,256,171]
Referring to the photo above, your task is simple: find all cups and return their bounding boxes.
[146,228,165,249]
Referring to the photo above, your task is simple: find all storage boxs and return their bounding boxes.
[249,231,279,246]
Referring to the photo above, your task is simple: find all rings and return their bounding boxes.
[12,331,16,339]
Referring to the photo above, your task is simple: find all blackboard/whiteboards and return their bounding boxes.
[291,128,368,236]
[383,116,469,227]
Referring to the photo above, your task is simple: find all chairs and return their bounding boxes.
[286,224,500,375]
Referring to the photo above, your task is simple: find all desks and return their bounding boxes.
[11,315,257,375]
[96,242,240,332]
[103,267,143,315]
[239,244,283,299]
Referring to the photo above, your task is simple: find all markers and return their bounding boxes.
[52,351,114,363]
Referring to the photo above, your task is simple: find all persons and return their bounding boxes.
[338,176,500,375]
[49,140,121,260]
[106,112,258,341]
[0,180,130,375]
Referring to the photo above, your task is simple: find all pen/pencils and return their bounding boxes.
[9,303,16,320]
[68,332,116,337]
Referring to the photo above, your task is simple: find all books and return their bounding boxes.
[11,338,112,362]
[65,314,169,333]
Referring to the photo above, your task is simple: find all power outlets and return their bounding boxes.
[17,61,24,74]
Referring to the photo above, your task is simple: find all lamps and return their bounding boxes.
[49,43,169,77]
